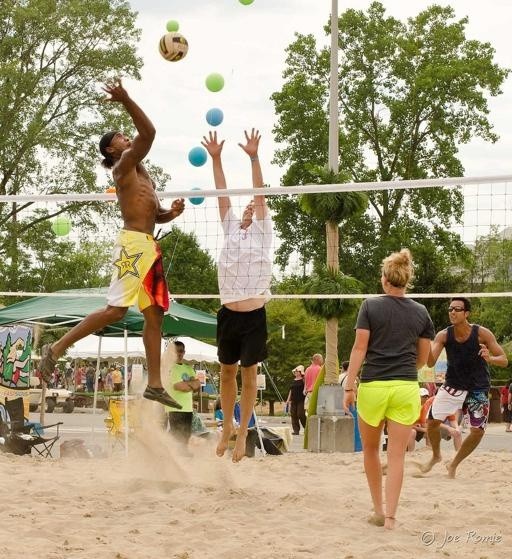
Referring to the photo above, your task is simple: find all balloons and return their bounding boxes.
[239,0,254,5]
[189,147,207,167]
[105,188,117,202]
[206,108,224,126]
[52,217,71,236]
[188,188,204,205]
[166,20,179,32]
[205,72,224,92]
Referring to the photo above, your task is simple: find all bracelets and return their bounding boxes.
[250,155,259,161]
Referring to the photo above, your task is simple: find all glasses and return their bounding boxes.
[446,306,466,312]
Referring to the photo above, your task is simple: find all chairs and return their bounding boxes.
[108,399,153,451]
[215,399,290,456]
[4,397,63,459]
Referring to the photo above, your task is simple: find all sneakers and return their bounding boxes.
[142,384,183,410]
[38,342,57,383]
[292,431,300,435]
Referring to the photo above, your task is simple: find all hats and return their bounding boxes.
[291,364,305,376]
[420,388,429,397]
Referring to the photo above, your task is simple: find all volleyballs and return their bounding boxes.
[157,32,189,62]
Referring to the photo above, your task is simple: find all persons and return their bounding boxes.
[508,383,512,432]
[499,381,510,432]
[166,342,201,456]
[285,354,465,457]
[343,248,435,531]
[37,75,183,409]
[419,298,508,478]
[33,360,133,392]
[201,127,274,463]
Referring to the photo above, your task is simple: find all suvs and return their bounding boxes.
[27,377,75,413]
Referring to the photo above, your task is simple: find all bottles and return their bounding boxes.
[285,404,288,413]
